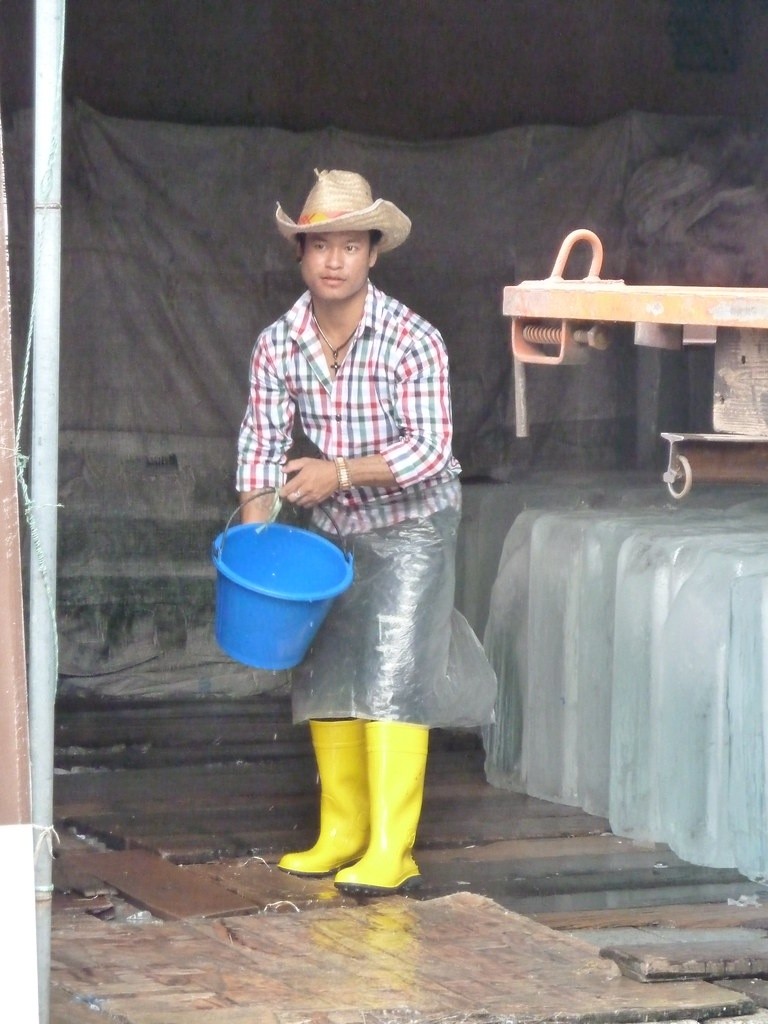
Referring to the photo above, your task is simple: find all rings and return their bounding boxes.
[294,489,302,498]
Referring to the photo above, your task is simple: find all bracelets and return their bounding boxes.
[334,456,352,494]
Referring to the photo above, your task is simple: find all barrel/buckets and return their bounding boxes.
[212,488,355,672]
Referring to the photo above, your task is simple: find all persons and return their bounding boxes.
[234,168,497,898]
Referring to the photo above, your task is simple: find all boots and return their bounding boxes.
[335,722,428,895]
[278,720,368,877]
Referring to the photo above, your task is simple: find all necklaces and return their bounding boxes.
[312,313,358,376]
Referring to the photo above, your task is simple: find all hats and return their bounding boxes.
[275,166,412,252]
